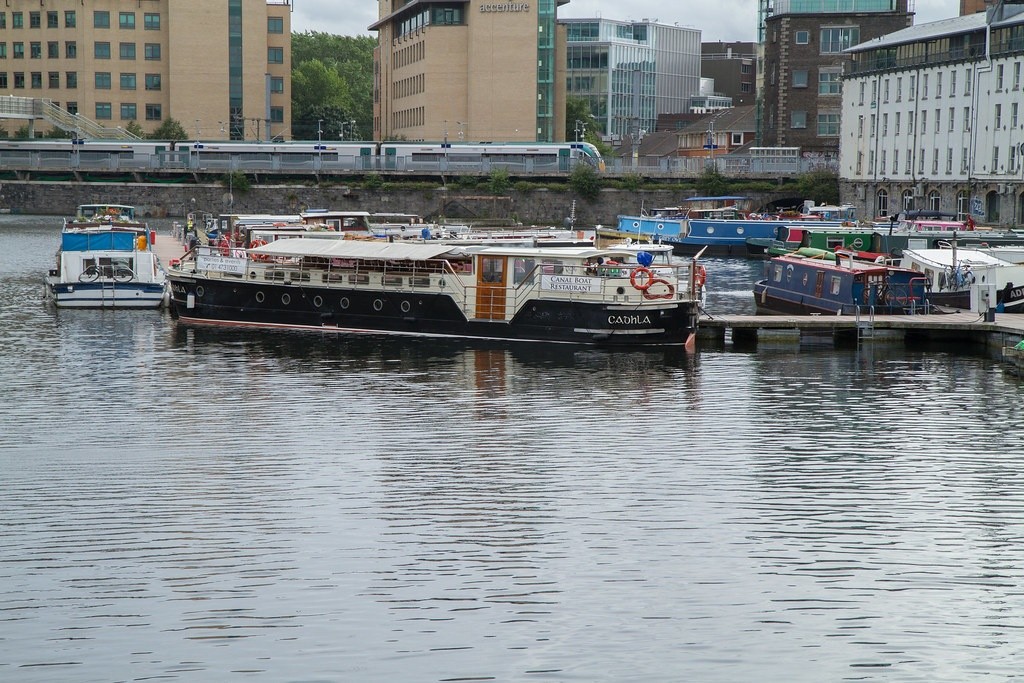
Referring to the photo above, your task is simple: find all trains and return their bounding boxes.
[0,138,607,184]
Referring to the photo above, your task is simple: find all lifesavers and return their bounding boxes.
[641,278,676,301]
[247,239,270,262]
[697,264,708,288]
[629,265,654,292]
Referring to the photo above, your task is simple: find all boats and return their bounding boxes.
[897,247,1024,313]
[43,202,171,310]
[164,197,1024,262]
[163,235,706,345]
[753,247,929,317]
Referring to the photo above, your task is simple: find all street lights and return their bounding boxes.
[349,120,356,140]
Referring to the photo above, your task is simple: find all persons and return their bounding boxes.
[597,257,604,276]
